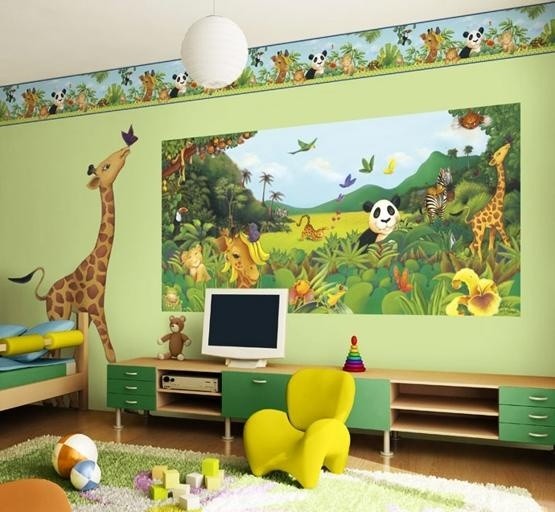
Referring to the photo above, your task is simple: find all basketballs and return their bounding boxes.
[70,461,102,491]
[52,432,98,479]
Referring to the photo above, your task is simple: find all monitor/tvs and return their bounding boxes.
[200,287,290,369]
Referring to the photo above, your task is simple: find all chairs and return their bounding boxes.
[244,366,359,493]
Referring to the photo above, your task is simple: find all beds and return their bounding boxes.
[0,310,92,421]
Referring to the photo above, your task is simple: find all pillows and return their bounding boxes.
[0,319,77,363]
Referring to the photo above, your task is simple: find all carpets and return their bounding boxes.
[0,435,548,512]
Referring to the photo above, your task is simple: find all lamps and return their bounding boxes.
[180,0,249,90]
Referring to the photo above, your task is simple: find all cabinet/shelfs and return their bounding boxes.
[106,354,553,466]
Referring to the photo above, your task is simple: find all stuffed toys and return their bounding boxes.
[157,314,191,361]
